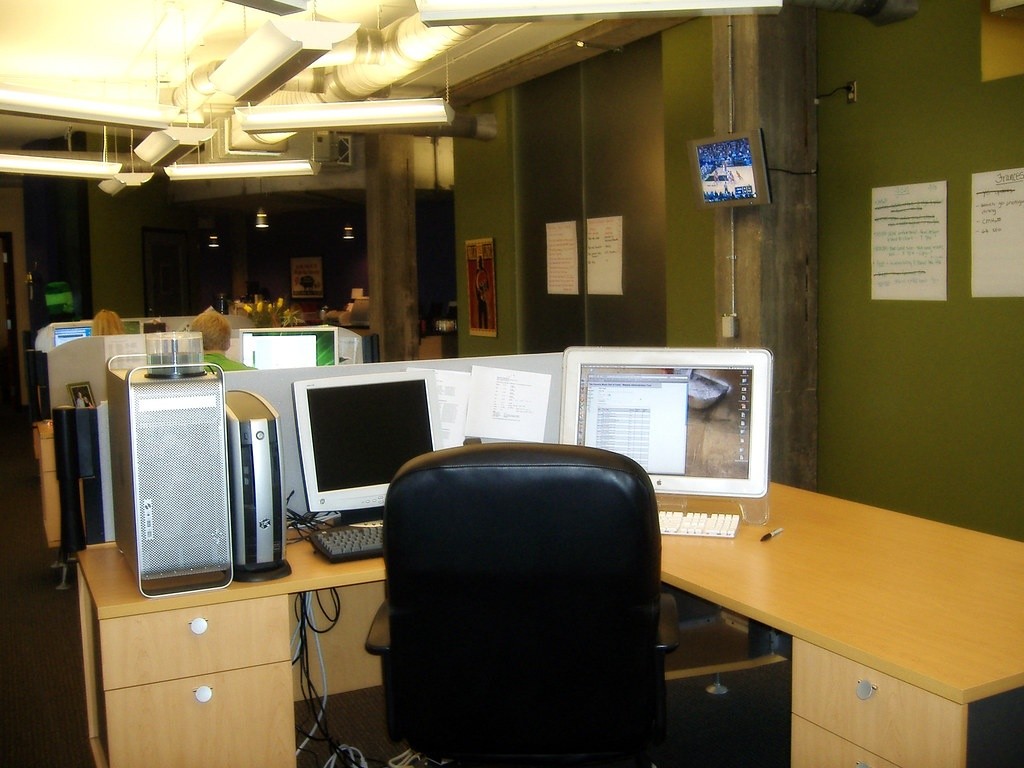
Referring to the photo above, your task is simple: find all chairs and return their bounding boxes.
[364,436,666,767]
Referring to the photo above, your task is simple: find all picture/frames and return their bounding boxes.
[464,237,498,339]
[290,255,325,298]
[68,380,95,410]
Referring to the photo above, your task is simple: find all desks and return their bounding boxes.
[75,483,1024,768]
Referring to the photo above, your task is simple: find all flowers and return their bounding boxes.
[243,298,299,327]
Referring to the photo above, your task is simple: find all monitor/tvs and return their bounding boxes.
[689,130,772,210]
[292,369,447,525]
[239,328,338,370]
[558,346,774,523]
[35,319,144,354]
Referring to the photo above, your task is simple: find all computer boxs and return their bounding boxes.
[102,354,234,598]
[221,389,285,571]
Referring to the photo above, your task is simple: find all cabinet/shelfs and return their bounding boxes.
[81,580,296,768]
[793,638,1024,768]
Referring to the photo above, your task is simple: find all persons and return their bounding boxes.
[697,138,757,202]
[186,310,258,372]
[90,308,127,336]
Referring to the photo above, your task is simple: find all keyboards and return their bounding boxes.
[308,519,383,564]
[657,510,740,539]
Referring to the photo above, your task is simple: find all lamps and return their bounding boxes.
[415,0,784,29]
[209,232,220,247]
[344,220,355,240]
[163,134,323,181]
[0,125,122,178]
[255,180,272,228]
[234,52,456,135]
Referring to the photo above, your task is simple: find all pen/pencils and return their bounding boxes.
[760,527,783,540]
